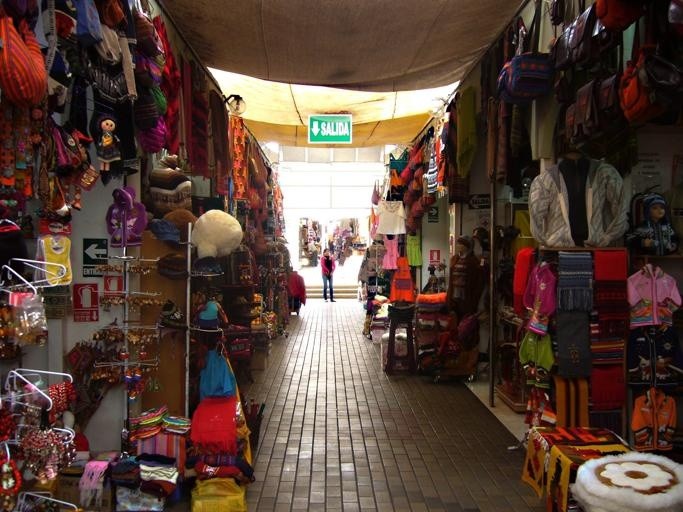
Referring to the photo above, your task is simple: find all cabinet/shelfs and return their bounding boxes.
[494,309,538,413]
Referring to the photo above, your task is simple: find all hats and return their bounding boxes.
[152,209,229,332]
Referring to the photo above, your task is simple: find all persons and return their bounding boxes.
[528,152,630,248]
[62,120,93,211]
[446,234,485,364]
[305,241,318,266]
[625,191,679,256]
[321,249,336,302]
[47,85,67,113]
[97,113,121,177]
[41,32,73,87]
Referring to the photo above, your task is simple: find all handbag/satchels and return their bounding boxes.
[48,0,171,154]
[1,0,47,107]
[495,1,665,165]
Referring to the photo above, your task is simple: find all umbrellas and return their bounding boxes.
[64,340,122,434]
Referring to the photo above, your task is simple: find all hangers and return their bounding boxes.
[537,139,614,178]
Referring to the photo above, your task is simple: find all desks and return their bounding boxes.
[386,320,417,375]
